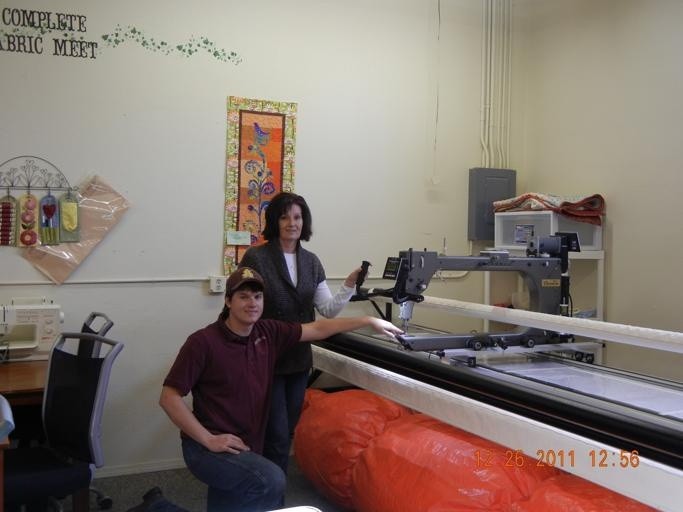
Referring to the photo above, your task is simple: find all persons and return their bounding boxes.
[239,192,369,476]
[123,266,404,512]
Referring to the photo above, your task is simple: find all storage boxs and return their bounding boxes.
[494,210,603,252]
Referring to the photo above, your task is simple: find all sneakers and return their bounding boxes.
[126,487,162,512]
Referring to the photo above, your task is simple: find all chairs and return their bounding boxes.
[1,310,124,512]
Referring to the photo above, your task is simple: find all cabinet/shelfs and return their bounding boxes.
[482,248,606,365]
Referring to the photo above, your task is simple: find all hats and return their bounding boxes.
[226,267,265,290]
